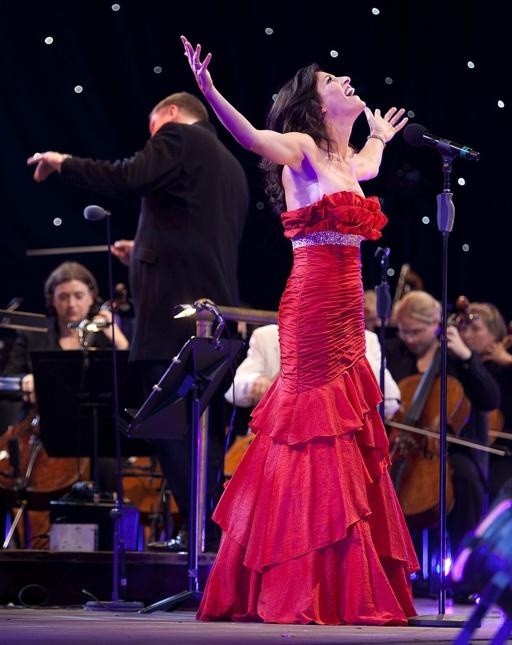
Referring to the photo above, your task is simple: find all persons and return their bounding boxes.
[181,34,420,625]
[27,92,253,553]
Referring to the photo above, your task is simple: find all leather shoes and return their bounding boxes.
[145,527,190,553]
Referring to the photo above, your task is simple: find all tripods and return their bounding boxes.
[136,413,208,614]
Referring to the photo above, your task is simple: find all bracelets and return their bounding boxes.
[367,134,386,147]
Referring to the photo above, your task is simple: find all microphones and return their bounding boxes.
[402,121,481,161]
[83,205,115,222]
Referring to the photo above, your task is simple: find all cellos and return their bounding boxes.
[387,295,470,529]
[0,285,130,548]
